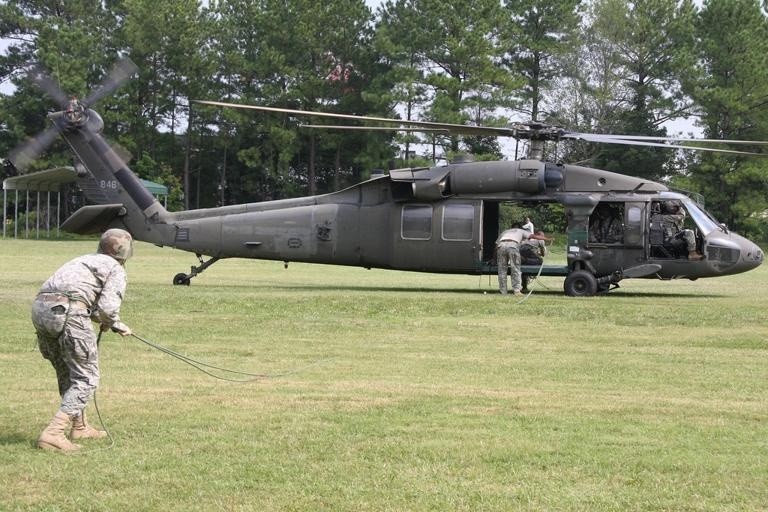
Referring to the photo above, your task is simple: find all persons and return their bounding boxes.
[495,223,553,295]
[31,228,133,454]
[521,217,535,234]
[657,201,702,259]
[519,231,545,294]
[588,204,624,244]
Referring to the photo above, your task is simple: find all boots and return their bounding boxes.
[38,412,77,451]
[71,410,107,439]
[688,251,702,259]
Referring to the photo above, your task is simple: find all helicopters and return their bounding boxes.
[2,55,768,296]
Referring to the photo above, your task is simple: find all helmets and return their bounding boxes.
[97,228,133,260]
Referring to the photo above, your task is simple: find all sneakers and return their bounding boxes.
[514,292,523,295]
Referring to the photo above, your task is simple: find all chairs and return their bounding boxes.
[649,203,680,259]
